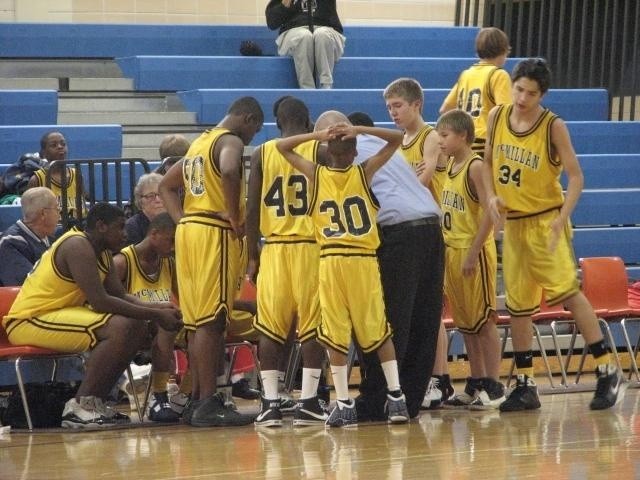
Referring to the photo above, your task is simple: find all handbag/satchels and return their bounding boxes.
[240,39,275,56]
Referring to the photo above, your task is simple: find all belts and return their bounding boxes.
[382,216,440,231]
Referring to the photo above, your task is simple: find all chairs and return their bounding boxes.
[441,283,555,396]
[508,289,574,393]
[568,256,638,389]
[1,285,92,432]
[173,336,257,404]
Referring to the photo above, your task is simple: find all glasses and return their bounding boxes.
[140,192,163,201]
[41,205,61,213]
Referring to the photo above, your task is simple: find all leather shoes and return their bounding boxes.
[353,396,386,422]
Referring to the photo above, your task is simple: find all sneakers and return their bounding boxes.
[146,392,179,422]
[277,393,298,411]
[499,375,541,411]
[191,393,253,427]
[444,378,483,409]
[217,386,238,411]
[470,379,506,410]
[589,365,627,410]
[83,399,131,426]
[441,374,455,402]
[384,393,409,423]
[420,376,448,410]
[61,396,116,430]
[293,397,327,426]
[233,379,259,399]
[183,400,206,424]
[165,378,181,402]
[254,397,282,427]
[171,392,191,415]
[325,399,357,426]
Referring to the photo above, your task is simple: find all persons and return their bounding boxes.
[0,186,62,428]
[27,132,94,240]
[440,29,517,270]
[266,0,348,89]
[275,95,316,134]
[483,59,629,412]
[347,111,445,429]
[2,204,184,433]
[245,100,330,428]
[113,214,193,422]
[350,113,377,129]
[275,123,408,429]
[382,78,453,414]
[149,135,192,176]
[434,110,509,414]
[125,172,180,256]
[162,97,265,429]
